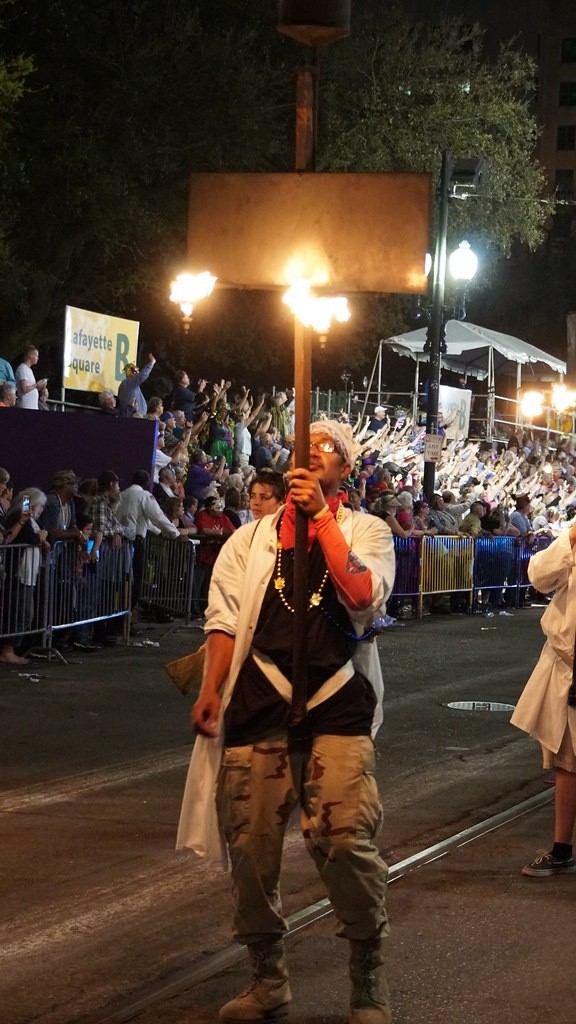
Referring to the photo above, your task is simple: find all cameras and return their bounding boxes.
[213,455,221,461]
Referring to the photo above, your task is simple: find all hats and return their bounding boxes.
[99,470,125,481]
[380,495,402,508]
[124,364,141,378]
[511,491,532,509]
[53,470,82,486]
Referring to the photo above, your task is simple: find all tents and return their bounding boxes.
[378,320,566,402]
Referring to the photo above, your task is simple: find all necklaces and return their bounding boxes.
[274,501,342,613]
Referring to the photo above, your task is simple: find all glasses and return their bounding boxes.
[309,441,336,455]
[422,504,431,509]
[436,497,443,500]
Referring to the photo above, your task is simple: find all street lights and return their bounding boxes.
[423,238,479,508]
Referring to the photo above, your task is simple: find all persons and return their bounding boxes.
[175,422,395,1024]
[0,342,576,665]
[248,469,292,520]
[509,518,576,877]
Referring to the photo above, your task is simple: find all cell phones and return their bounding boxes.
[21,495,31,514]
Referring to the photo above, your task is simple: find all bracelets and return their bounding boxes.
[18,520,23,526]
[313,504,329,519]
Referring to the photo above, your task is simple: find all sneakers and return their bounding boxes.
[522,845,576,875]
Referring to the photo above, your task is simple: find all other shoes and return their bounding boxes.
[223,982,292,1024]
[141,605,177,626]
[498,608,516,617]
[346,983,392,1023]
[128,623,144,637]
